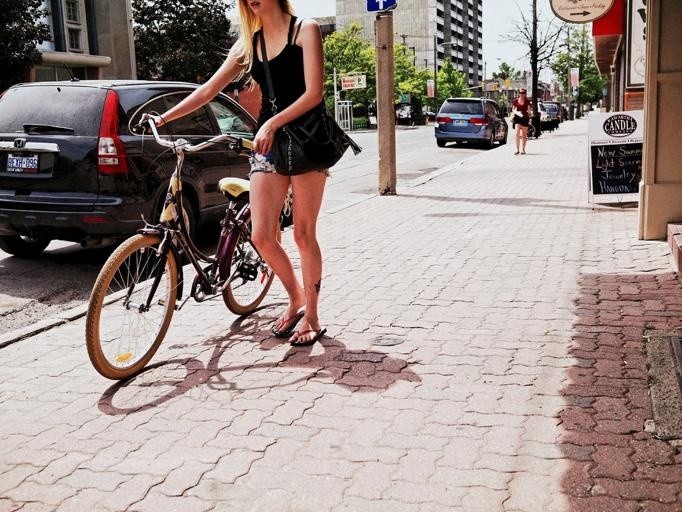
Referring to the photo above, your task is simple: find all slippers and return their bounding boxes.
[271,310,304,337]
[291,328,327,347]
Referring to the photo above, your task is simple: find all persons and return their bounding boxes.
[511,87,531,155]
[137,0,339,347]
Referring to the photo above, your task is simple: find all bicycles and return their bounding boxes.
[85,113,281,379]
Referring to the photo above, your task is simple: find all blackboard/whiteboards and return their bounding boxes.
[590,143,642,195]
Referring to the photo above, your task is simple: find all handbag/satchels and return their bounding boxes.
[273,106,362,176]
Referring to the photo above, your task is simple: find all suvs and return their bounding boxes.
[434,97,508,148]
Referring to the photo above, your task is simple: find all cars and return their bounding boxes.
[529,99,564,124]
[0,63,293,259]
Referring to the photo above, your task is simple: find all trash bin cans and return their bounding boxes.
[413,112,426,125]
[583,104,590,112]
[367,111,376,128]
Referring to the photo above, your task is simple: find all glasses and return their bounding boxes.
[519,91,525,94]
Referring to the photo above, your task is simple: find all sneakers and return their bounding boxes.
[514,151,525,155]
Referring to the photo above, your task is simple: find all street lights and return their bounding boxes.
[433,35,456,97]
